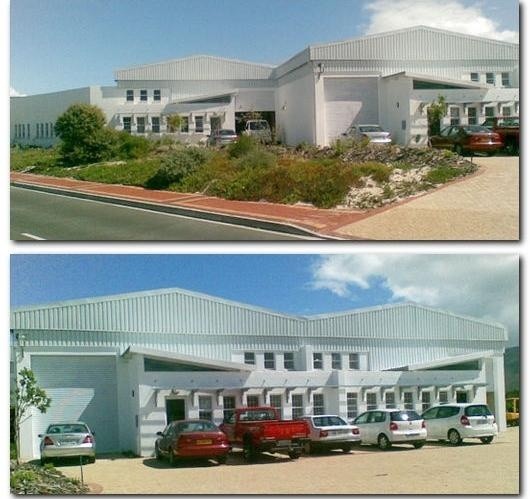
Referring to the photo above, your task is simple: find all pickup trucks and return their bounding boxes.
[219,406,310,460]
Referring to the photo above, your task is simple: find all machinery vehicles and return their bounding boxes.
[507,396,519,427]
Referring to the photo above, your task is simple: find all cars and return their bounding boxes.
[297,414,364,454]
[152,419,229,464]
[341,124,394,145]
[208,127,239,147]
[351,408,428,451]
[36,421,97,463]
[430,123,503,156]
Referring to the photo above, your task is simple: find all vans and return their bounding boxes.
[242,116,272,146]
[481,114,520,153]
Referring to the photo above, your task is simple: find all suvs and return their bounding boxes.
[422,402,497,446]
